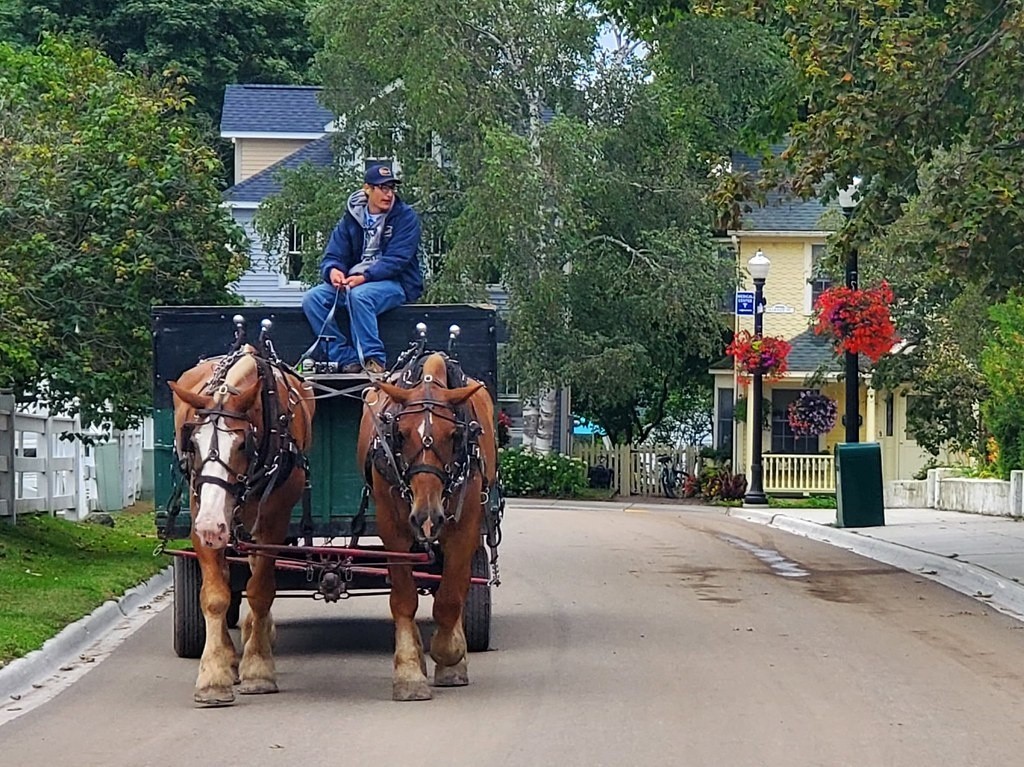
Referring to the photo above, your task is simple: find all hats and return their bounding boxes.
[364,165,402,184]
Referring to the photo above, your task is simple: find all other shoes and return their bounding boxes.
[362,360,384,374]
[343,363,361,373]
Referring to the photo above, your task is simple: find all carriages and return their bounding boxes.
[145,302,511,704]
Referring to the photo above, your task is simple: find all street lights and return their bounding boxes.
[744,249,771,504]
[839,175,866,444]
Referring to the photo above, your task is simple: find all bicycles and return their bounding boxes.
[657,439,692,499]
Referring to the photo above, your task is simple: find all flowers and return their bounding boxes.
[725,329,795,383]
[789,391,839,439]
[809,279,901,361]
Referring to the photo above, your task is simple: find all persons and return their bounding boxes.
[301,165,423,373]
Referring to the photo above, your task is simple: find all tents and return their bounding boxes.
[572,412,607,447]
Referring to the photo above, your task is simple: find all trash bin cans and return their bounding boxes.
[833,442,885,528]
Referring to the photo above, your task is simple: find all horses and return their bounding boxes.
[168,343,315,707]
[357,352,497,703]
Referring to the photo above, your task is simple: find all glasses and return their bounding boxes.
[373,184,399,193]
